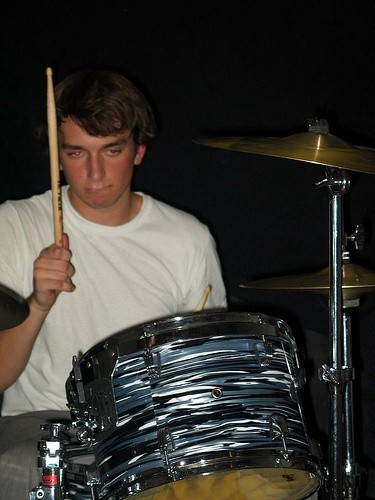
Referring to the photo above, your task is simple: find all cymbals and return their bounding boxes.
[237,233,375,299]
[194,114,375,183]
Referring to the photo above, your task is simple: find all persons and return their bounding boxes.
[0,69,228,500]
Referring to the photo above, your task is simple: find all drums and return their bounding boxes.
[65,306,324,500]
